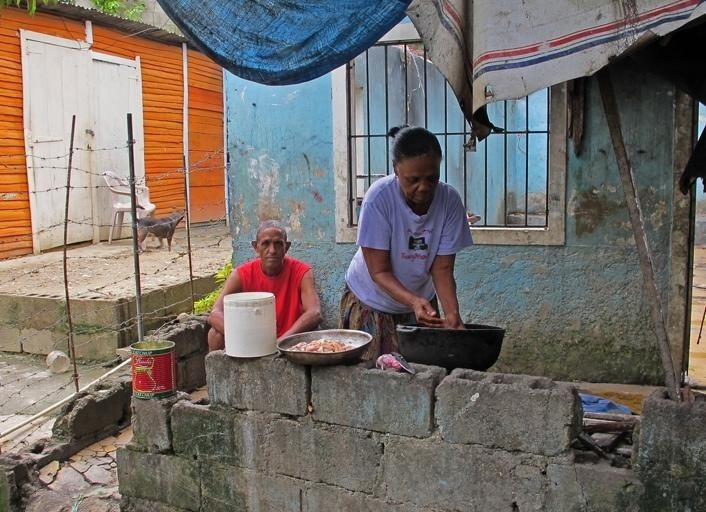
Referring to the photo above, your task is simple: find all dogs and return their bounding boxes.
[132,204,188,253]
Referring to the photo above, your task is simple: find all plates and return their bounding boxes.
[277,328,374,366]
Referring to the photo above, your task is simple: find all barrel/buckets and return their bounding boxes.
[130,341,177,400]
[223,291,278,358]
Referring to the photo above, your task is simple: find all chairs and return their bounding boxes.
[102,169,159,254]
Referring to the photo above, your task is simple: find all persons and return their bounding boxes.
[336,123,474,366]
[207,218,324,354]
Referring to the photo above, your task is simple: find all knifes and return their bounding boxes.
[391,351,415,375]
